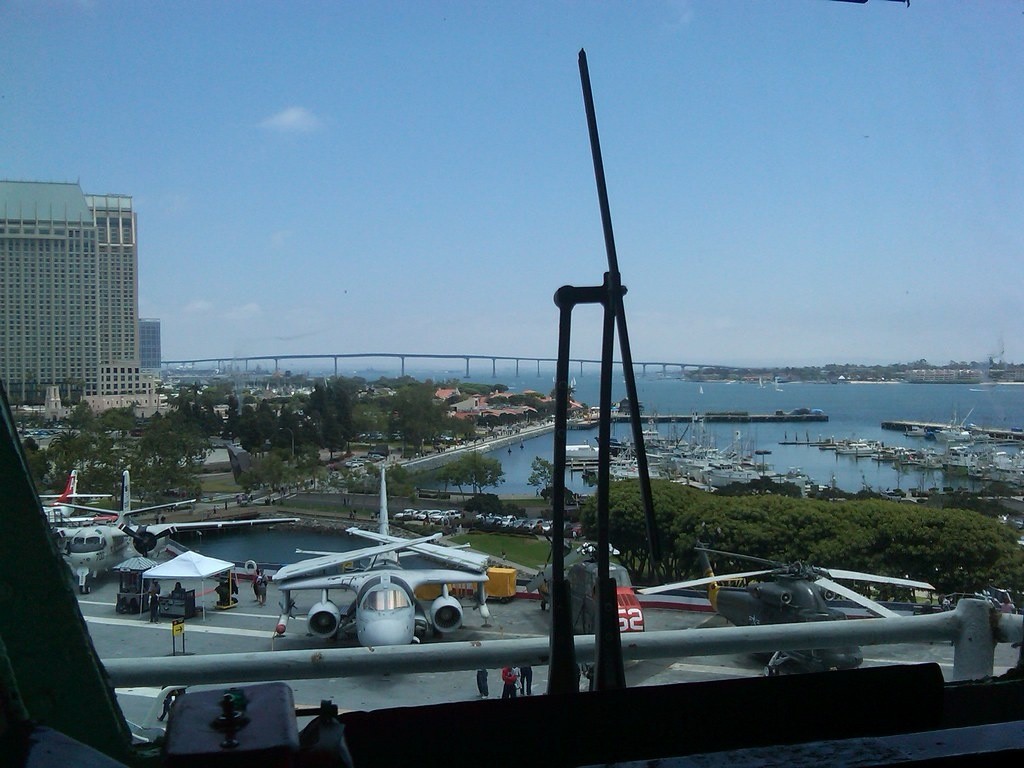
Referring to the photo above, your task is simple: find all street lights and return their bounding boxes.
[279,426,295,461]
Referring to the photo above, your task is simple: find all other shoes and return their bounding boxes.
[262,602,266,606]
[477,694,483,697]
[482,696,488,699]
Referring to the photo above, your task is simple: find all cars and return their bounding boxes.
[475,512,554,532]
[345,453,386,468]
[394,508,462,526]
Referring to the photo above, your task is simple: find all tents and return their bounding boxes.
[137,550,237,622]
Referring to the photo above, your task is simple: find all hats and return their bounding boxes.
[153,580,159,583]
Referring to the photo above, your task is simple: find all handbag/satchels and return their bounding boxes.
[514,677,522,689]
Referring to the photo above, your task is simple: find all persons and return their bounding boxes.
[250,568,269,605]
[474,668,489,699]
[499,664,532,699]
[146,580,162,624]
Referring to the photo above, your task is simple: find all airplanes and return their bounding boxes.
[273,462,491,649]
[526,536,644,638]
[37,470,302,594]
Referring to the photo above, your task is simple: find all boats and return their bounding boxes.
[835,406,1024,489]
[565,409,828,496]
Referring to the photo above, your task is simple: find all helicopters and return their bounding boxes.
[638,540,944,678]
